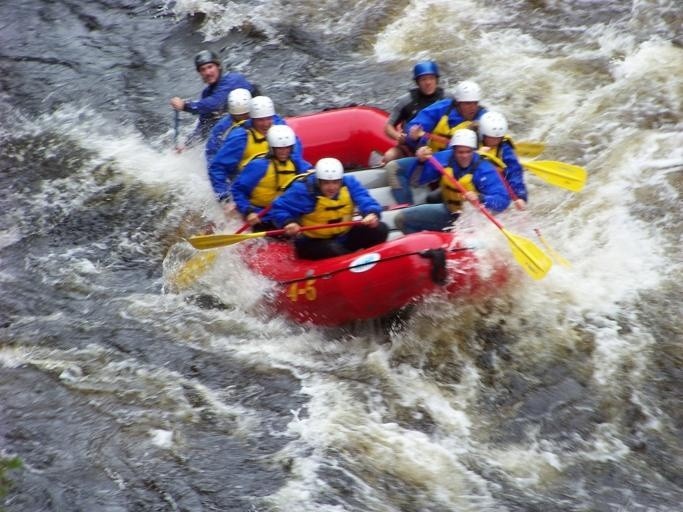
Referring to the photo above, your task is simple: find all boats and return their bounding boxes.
[220,107,514,328]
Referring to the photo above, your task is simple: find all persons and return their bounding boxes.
[206,88,288,167]
[169,50,261,156]
[393,128,512,236]
[379,58,458,166]
[207,96,303,220]
[446,112,530,210]
[263,158,393,264]
[406,82,516,155]
[232,123,319,226]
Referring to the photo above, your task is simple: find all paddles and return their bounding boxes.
[498,168,571,271]
[174,199,274,287]
[399,132,543,159]
[190,218,376,248]
[429,154,550,282]
[413,125,589,191]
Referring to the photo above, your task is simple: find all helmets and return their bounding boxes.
[448,127,478,150]
[452,80,482,104]
[264,124,297,149]
[314,157,345,182]
[226,86,253,116]
[193,49,221,71]
[412,62,440,81]
[248,94,276,120]
[477,110,509,142]
